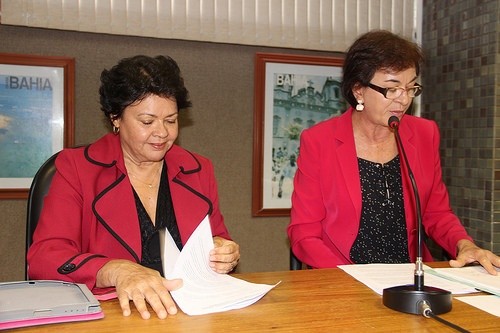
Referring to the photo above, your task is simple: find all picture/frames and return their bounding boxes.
[251,52,345,217]
[0,51,76,200]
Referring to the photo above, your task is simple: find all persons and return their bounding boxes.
[26,55,241,320]
[287,30,500,276]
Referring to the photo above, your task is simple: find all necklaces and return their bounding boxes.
[125,163,163,188]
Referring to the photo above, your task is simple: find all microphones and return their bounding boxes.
[382,116,454,315]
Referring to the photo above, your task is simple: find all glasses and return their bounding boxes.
[368,82,423,100]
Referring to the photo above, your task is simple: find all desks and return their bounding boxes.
[0,258,500,332]
